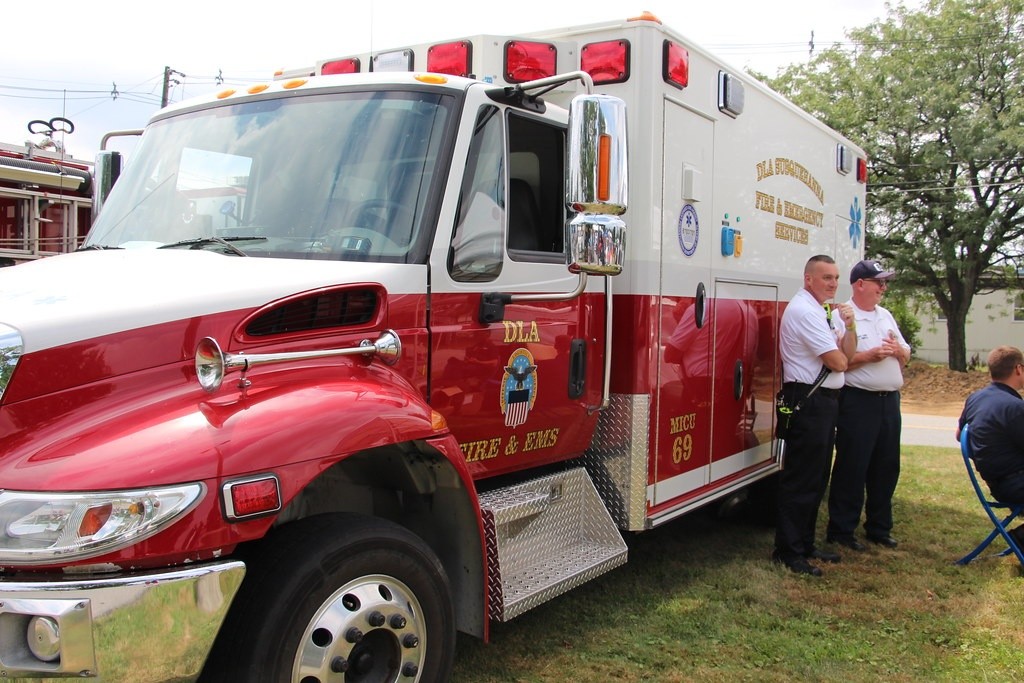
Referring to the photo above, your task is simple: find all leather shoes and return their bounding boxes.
[866,533,897,547]
[810,550,840,563]
[771,551,821,576]
[827,536,864,551]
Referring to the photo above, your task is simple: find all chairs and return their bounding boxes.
[950,423,1024,571]
[457,178,543,251]
[268,183,319,237]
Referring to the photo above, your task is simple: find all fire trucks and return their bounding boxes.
[0,115,249,270]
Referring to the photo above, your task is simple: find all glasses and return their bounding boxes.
[863,279,889,285]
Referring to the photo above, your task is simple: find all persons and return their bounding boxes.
[535,334,570,379]
[955,346,1024,577]
[775,254,857,576]
[663,298,758,423]
[828,260,911,554]
[462,327,501,373]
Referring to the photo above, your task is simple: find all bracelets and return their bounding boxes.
[845,321,856,331]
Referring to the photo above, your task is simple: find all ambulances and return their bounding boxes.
[1,5,869,683]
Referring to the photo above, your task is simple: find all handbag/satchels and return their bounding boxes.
[774,407,792,440]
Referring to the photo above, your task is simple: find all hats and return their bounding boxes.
[850,260,895,284]
